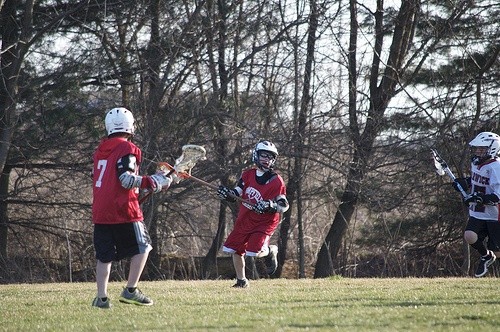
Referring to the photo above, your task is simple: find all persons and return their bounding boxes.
[91,107,172,310]
[451,132,500,277]
[217,141,290,289]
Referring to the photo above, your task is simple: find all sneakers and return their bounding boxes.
[231,278,250,288]
[118,287,154,305]
[474,250,496,277]
[92,297,110,309]
[265,244,278,275]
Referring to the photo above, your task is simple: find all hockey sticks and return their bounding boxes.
[138,145,206,206]
[156,161,257,207]
[430,147,468,198]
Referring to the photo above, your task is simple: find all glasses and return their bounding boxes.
[260,152,274,160]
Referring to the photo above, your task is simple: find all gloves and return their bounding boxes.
[216,185,236,201]
[150,169,173,194]
[453,176,472,192]
[252,201,270,214]
[462,191,485,209]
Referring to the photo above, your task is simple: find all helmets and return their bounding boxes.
[252,140,279,166]
[468,131,500,164]
[105,107,136,136]
